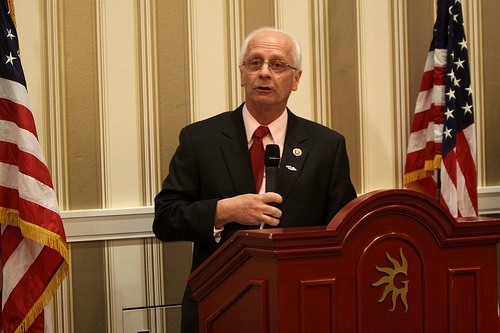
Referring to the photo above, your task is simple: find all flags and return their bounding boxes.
[400,0,483,223]
[0,0,74,333]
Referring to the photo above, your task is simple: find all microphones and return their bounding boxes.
[264,144,281,229]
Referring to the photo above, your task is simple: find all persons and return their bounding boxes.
[151,26,360,333]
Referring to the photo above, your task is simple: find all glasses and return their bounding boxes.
[242,58,297,73]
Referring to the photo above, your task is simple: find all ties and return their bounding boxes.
[249,125,270,194]
